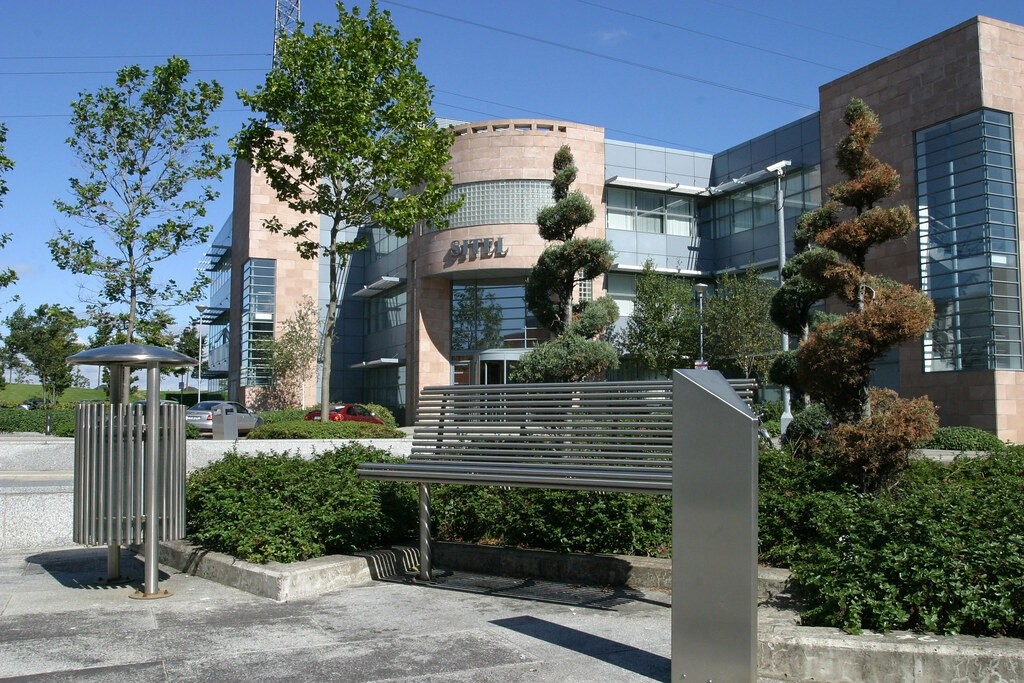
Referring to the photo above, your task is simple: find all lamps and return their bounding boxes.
[693,282,709,361]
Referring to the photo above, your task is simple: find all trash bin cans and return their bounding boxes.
[211,403,239,440]
[66,342,200,603]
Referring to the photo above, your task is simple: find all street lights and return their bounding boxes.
[196,305,208,402]
[693,283,708,371]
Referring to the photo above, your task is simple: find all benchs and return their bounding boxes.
[357,378,758,582]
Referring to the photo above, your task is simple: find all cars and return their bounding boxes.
[21,398,57,409]
[305,404,387,424]
[186,402,264,433]
